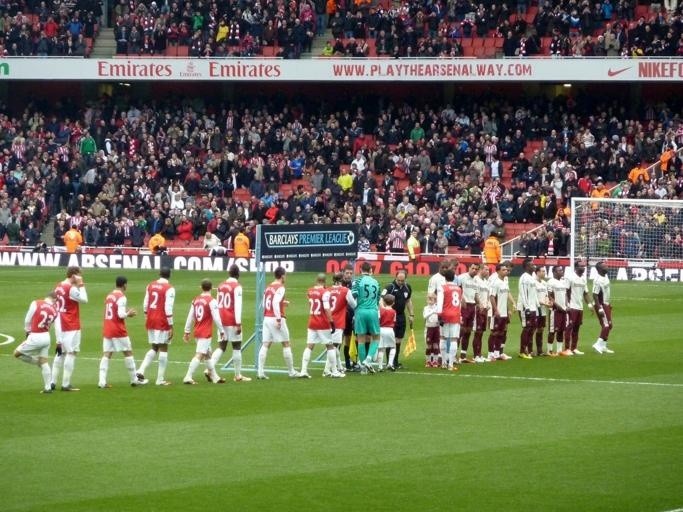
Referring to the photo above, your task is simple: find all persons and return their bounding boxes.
[0,1,682,265]
[13,265,252,393]
[423,259,613,370]
[258,266,415,379]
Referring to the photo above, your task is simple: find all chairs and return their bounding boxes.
[0,0,657,57]
[0,104,679,258]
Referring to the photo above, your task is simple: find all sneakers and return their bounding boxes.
[40,381,80,392]
[288,369,311,378]
[425,339,512,371]
[518,347,583,359]
[592,338,614,354]
[96,383,111,389]
[323,357,404,377]
[130,372,172,386]
[183,369,269,383]
